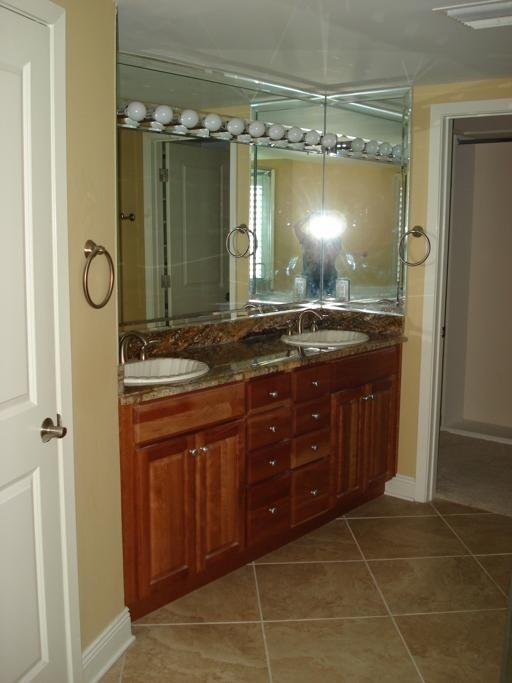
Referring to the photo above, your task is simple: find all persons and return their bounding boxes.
[292,209,341,297]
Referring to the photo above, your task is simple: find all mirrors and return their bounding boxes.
[117,50,414,333]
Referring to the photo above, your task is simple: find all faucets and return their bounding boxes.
[120,330,148,364]
[240,303,264,314]
[295,309,322,335]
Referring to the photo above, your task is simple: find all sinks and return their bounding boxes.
[280,328,369,346]
[119,354,210,386]
